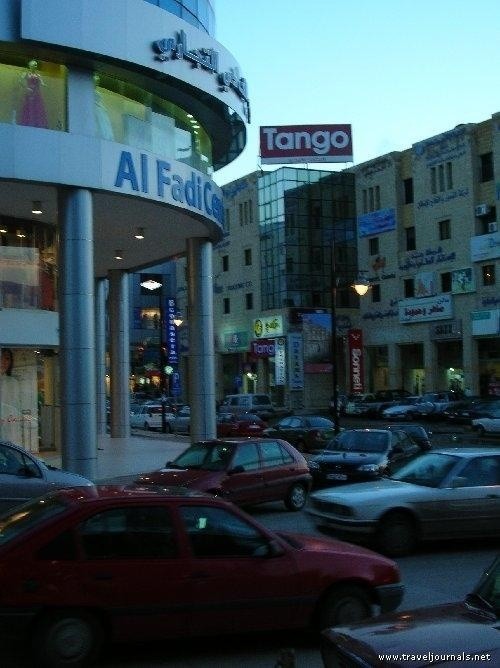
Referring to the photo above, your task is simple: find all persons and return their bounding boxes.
[16,58,50,128]
[0,348,22,445]
[93,76,116,141]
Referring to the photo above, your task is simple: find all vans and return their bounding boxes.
[221,392,271,417]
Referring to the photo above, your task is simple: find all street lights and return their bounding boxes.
[330,270,372,431]
[156,312,182,431]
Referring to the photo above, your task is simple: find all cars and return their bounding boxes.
[137,438,310,512]
[1,482,404,668]
[318,388,500,434]
[321,555,499,667]
[262,417,345,450]
[130,401,269,438]
[309,423,432,488]
[306,447,500,556]
[0,441,99,515]
